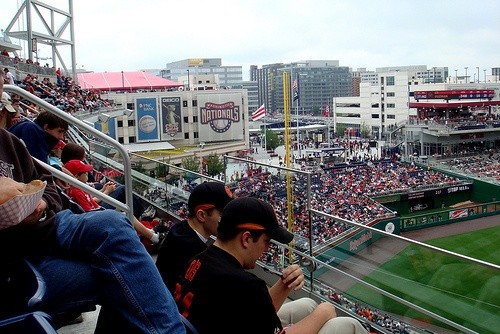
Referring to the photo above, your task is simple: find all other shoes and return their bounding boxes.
[144,206,156,218]
[151,219,164,234]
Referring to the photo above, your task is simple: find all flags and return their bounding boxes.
[293,80,299,101]
[251,104,265,121]
[324,104,329,116]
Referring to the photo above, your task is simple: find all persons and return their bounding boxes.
[322,289,410,334]
[0,69,186,334]
[0,48,190,255]
[421,112,500,183]
[184,134,461,268]
[170,197,368,334]
[155,181,236,290]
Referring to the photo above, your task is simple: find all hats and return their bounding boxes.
[0,91,17,113]
[219,197,294,244]
[64,160,93,174]
[187,181,235,211]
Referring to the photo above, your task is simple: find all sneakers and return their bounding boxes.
[151,230,171,250]
